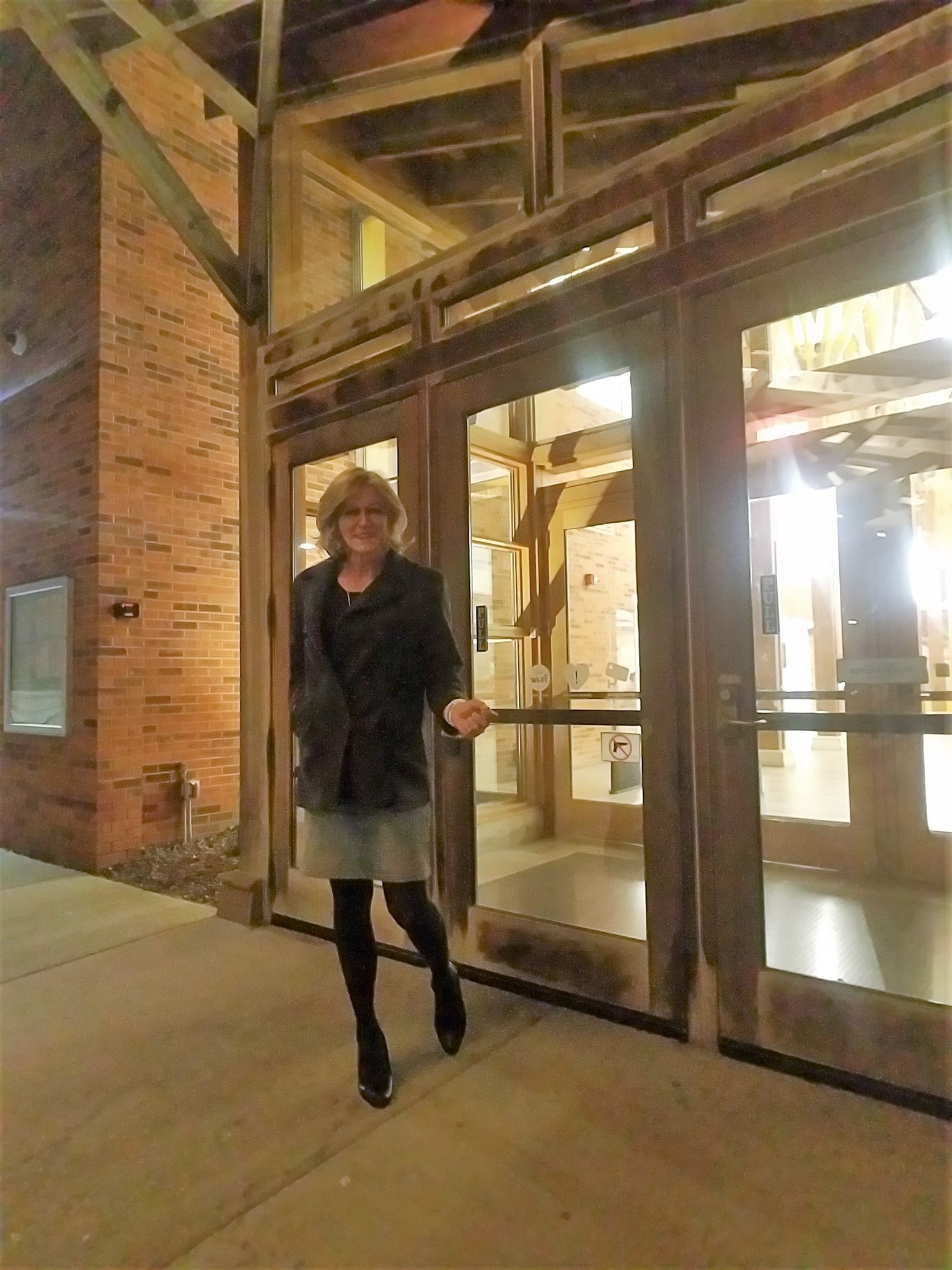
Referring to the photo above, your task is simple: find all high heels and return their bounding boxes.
[430,961,467,1057]
[356,1026,392,1108]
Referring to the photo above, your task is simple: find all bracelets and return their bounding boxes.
[448,702,458,728]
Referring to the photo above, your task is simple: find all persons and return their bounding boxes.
[287,467,499,1109]
